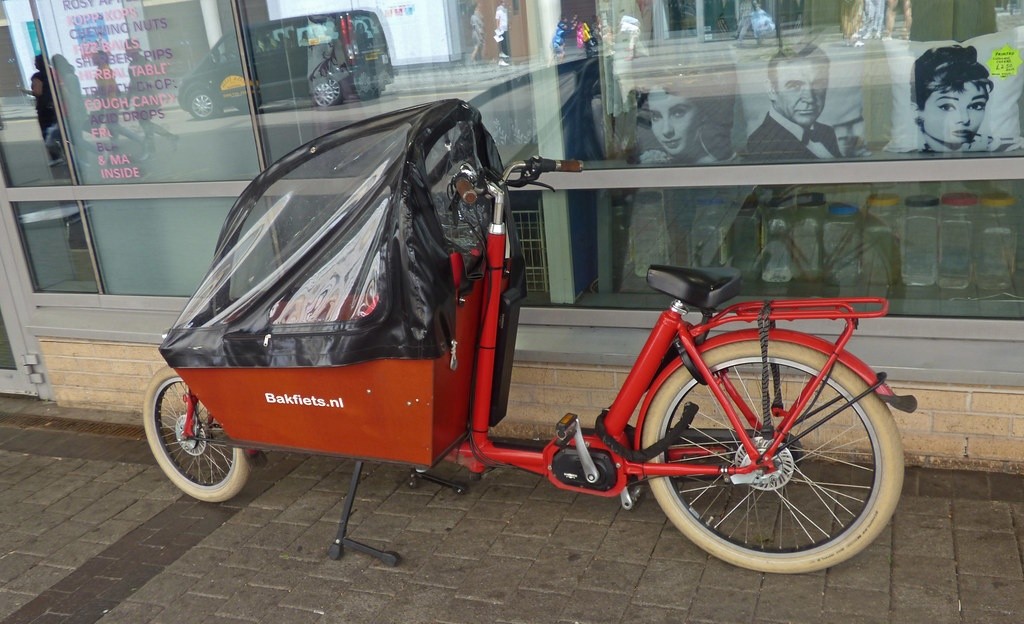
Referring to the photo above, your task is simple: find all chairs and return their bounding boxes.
[357,23,369,42]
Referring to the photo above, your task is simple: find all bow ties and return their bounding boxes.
[801,129,824,145]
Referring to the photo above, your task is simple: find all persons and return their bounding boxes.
[747,43,870,161]
[545,16,641,68]
[470,0,511,67]
[23,54,89,168]
[93,40,183,162]
[635,63,743,164]
[908,45,1024,153]
[852,0,912,48]
[734,0,765,44]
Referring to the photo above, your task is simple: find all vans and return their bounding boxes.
[176,10,395,122]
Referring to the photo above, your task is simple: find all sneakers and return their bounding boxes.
[499,52,509,58]
[498,59,509,66]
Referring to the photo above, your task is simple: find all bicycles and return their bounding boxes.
[412,56,905,319]
[144,97,917,575]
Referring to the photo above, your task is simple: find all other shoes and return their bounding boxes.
[170,134,181,153]
[47,158,64,167]
[854,41,864,48]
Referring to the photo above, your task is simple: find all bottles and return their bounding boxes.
[609,192,1016,292]
